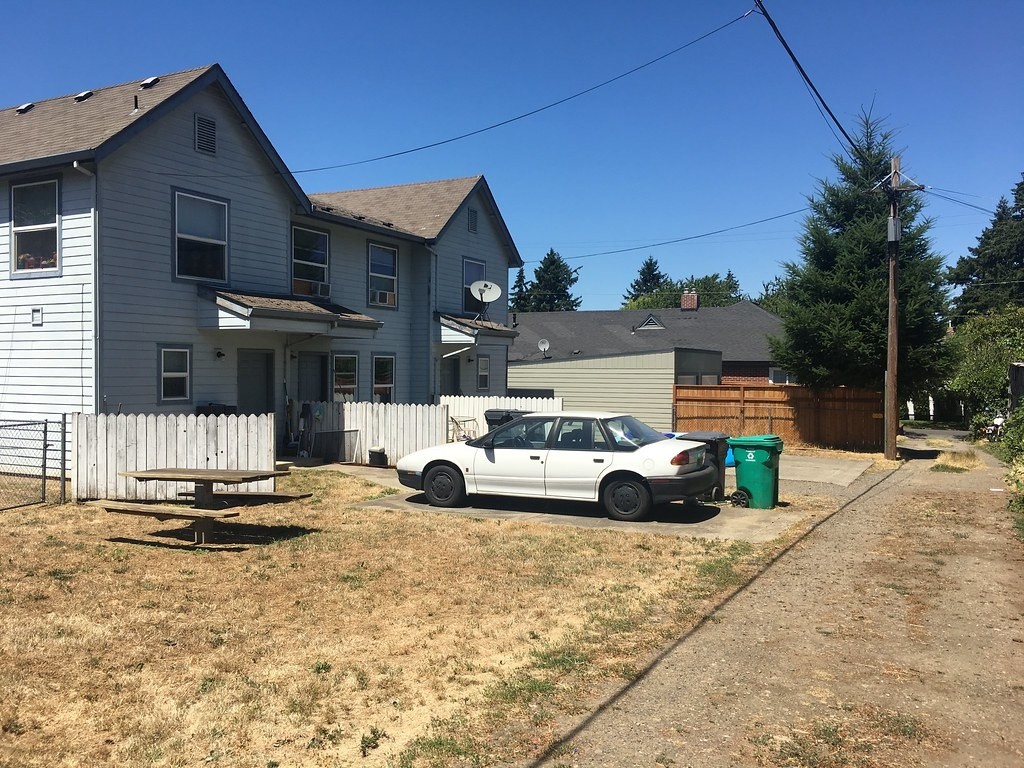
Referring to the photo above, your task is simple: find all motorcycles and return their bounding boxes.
[985,412,1008,443]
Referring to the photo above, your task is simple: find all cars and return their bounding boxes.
[396,411,717,522]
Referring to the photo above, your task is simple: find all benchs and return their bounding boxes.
[178,490,313,507]
[84,500,240,522]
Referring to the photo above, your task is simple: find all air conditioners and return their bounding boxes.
[311,284,330,298]
[370,291,389,305]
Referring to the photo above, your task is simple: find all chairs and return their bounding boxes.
[557,433,574,449]
[572,429,585,449]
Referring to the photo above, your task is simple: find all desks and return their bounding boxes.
[118,467,291,544]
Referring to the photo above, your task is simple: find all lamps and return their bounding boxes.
[290,352,298,362]
[215,348,226,362]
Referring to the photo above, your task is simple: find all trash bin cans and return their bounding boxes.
[724,434,784,509]
[676,431,731,501]
